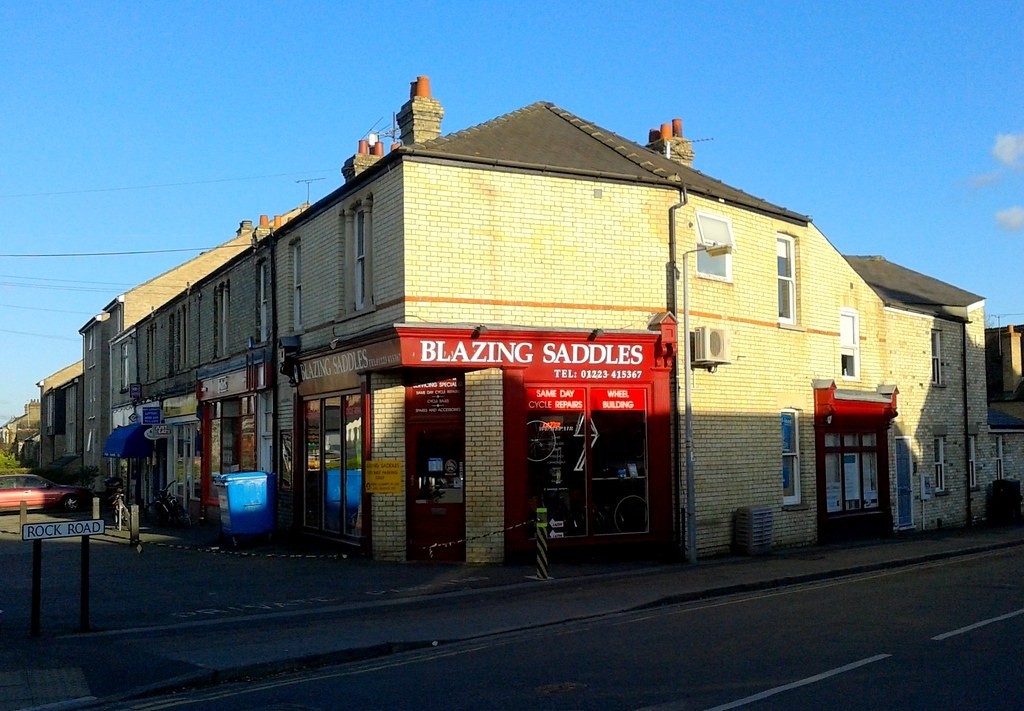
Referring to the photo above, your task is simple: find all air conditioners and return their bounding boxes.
[695,326,730,362]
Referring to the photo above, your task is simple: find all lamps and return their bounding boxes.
[591,329,605,338]
[476,324,489,334]
[329,338,340,350]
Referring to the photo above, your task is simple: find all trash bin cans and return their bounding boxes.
[992,478,1021,525]
[213,472,275,534]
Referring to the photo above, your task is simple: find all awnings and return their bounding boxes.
[103,423,155,458]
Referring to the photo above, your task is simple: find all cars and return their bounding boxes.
[0,474,91,514]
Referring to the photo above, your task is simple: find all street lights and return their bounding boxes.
[682,243,735,572]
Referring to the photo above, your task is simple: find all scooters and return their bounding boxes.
[108,484,132,531]
[155,479,192,532]
